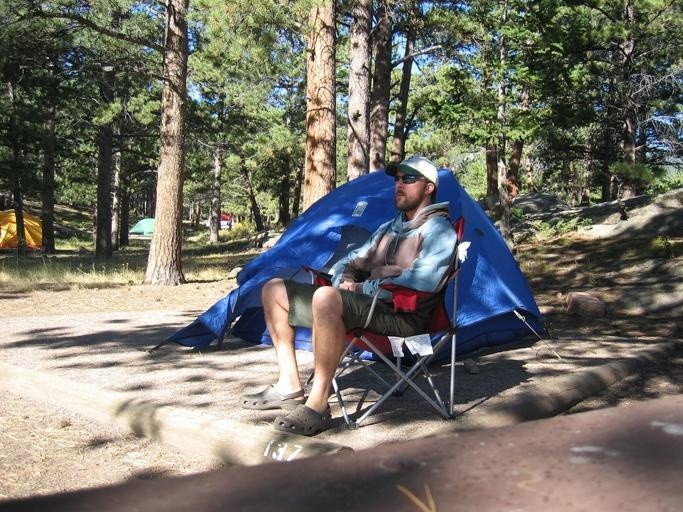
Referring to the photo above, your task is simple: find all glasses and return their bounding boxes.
[395,173,427,184]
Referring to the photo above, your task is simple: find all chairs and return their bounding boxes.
[302,216,466,433]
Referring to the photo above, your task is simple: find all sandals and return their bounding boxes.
[240,385,306,410]
[272,404,332,435]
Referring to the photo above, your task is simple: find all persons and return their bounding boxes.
[240,156,457,435]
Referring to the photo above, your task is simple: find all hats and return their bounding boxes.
[386,155,439,188]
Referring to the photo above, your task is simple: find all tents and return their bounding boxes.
[0,209,43,250]
[146,169,546,368]
[129,218,155,235]
